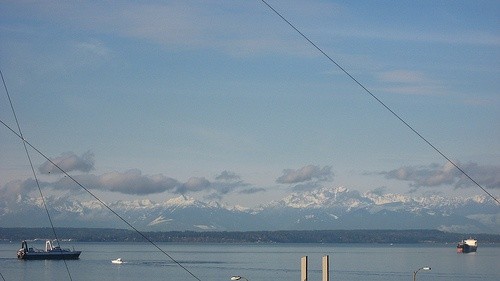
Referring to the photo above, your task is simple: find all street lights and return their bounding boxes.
[413,267,432,281]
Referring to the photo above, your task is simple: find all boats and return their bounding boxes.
[17,240,82,260]
[456,236,478,253]
[111,257,123,265]
